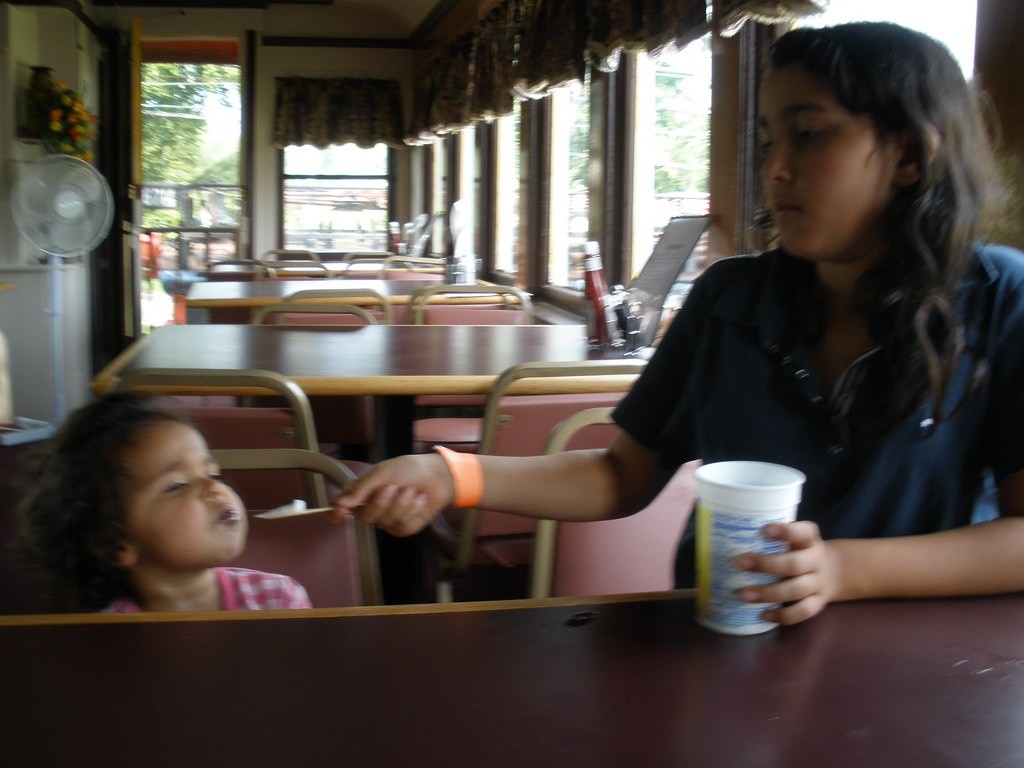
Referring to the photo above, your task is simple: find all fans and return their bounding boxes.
[0,149,114,446]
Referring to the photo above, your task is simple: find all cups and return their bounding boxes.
[690,461,807,636]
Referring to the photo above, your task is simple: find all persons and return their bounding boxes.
[16,389,314,613]
[330,21,1024,626]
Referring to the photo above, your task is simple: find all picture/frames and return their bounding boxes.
[39,85,99,165]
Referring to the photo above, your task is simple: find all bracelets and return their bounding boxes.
[434,445,485,508]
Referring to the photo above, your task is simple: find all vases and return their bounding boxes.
[25,63,56,138]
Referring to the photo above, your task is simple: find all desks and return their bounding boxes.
[187,279,534,308]
[0,594,1024,768]
[93,322,660,396]
[200,258,448,279]
[0,261,91,427]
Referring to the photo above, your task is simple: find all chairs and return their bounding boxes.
[0,250,1024,768]
